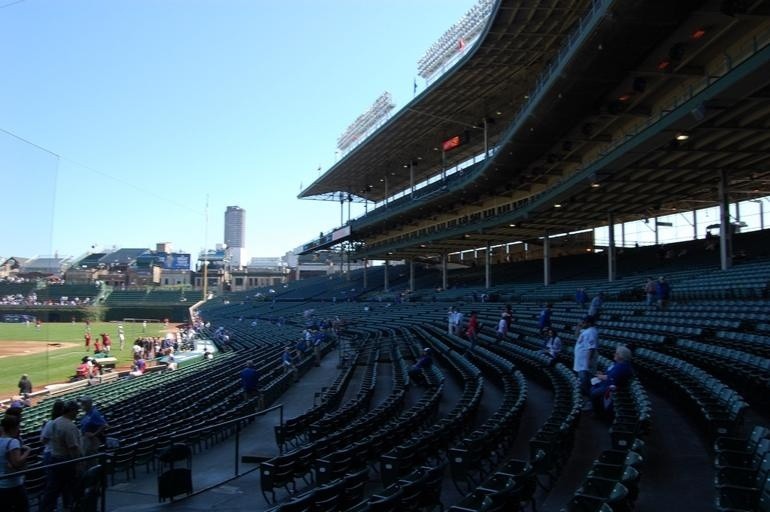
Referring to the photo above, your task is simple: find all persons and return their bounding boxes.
[75,318,233,378]
[17,374,32,395]
[224,282,288,326]
[332,282,502,313]
[536,275,671,418]
[282,309,347,384]
[447,306,513,354]
[1,392,109,512]
[409,347,433,386]
[1,275,105,328]
[240,359,261,424]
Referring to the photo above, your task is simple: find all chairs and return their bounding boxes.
[346,1,769,248]
[0,258,770,511]
